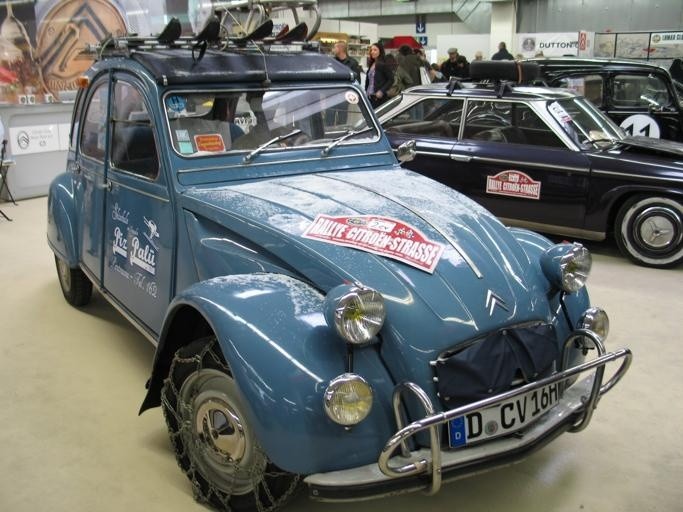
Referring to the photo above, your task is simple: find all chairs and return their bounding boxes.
[112,126,158,177]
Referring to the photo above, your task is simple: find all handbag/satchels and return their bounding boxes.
[420,66,430,85]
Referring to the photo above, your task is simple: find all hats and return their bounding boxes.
[448,48,457,54]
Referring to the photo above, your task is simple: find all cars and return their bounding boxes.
[45,16,631,512]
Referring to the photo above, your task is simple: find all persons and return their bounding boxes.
[668,57,683,101]
[321,40,516,129]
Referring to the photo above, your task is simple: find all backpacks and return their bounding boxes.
[386,72,401,98]
